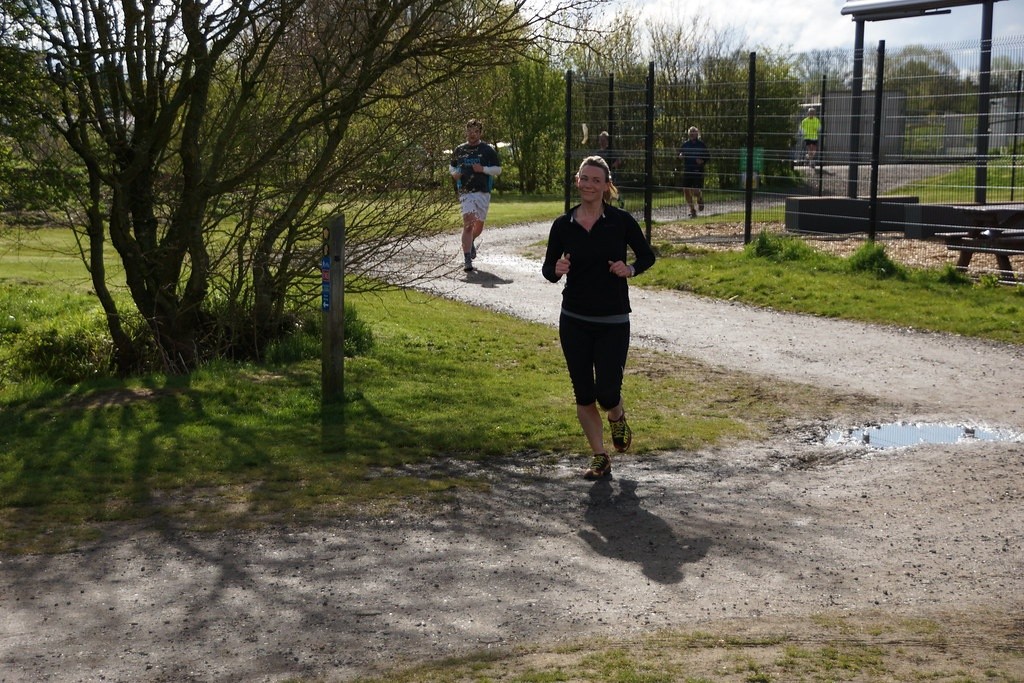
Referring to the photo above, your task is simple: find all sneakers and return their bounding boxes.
[583,455,611,478]
[608,408,632,452]
[464,242,477,271]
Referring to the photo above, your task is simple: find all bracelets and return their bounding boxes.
[627,265,634,278]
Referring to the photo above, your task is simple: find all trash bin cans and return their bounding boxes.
[740,170,760,191]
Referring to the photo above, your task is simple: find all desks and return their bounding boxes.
[955,226,1016,282]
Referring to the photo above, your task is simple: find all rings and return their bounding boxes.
[619,271,621,274]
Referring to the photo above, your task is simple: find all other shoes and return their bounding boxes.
[688,208,696,218]
[804,155,815,168]
[697,196,705,211]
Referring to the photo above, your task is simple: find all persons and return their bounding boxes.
[449,119,501,271]
[798,108,821,168]
[678,126,710,216]
[596,131,626,209]
[542,156,657,481]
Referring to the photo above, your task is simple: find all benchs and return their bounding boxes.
[947,245,1024,256]
[934,230,1024,249]
